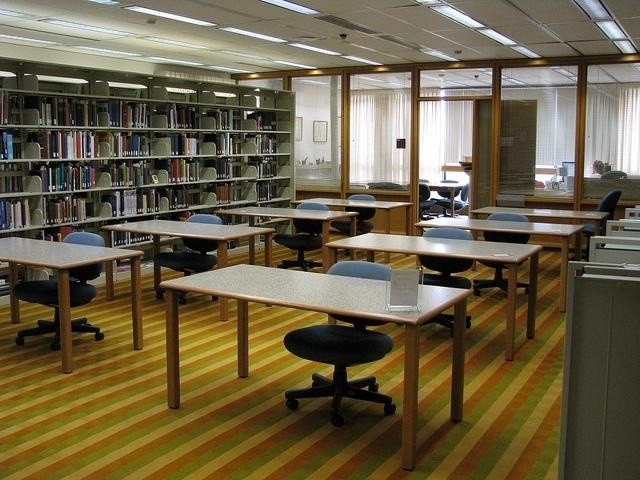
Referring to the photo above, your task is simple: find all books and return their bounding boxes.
[155,132,199,156]
[155,103,197,129]
[156,158,199,184]
[29,131,94,159]
[98,189,157,217]
[256,204,289,223]
[98,160,152,187]
[0,198,31,231]
[0,259,25,298]
[204,132,243,155]
[204,181,246,205]
[43,193,95,226]
[38,95,100,128]
[110,218,153,245]
[204,156,241,180]
[100,132,151,157]
[0,164,25,193]
[243,156,277,179]
[99,99,149,128]
[9,219,105,244]
[256,180,281,201]
[207,108,240,130]
[29,161,93,192]
[0,128,22,159]
[1,92,25,125]
[160,205,252,225]
[245,133,278,154]
[159,184,201,210]
[247,111,276,131]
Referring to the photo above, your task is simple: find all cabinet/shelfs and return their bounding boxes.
[1,55,308,303]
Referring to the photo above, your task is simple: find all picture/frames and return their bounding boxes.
[295,116,303,142]
[312,120,328,143]
